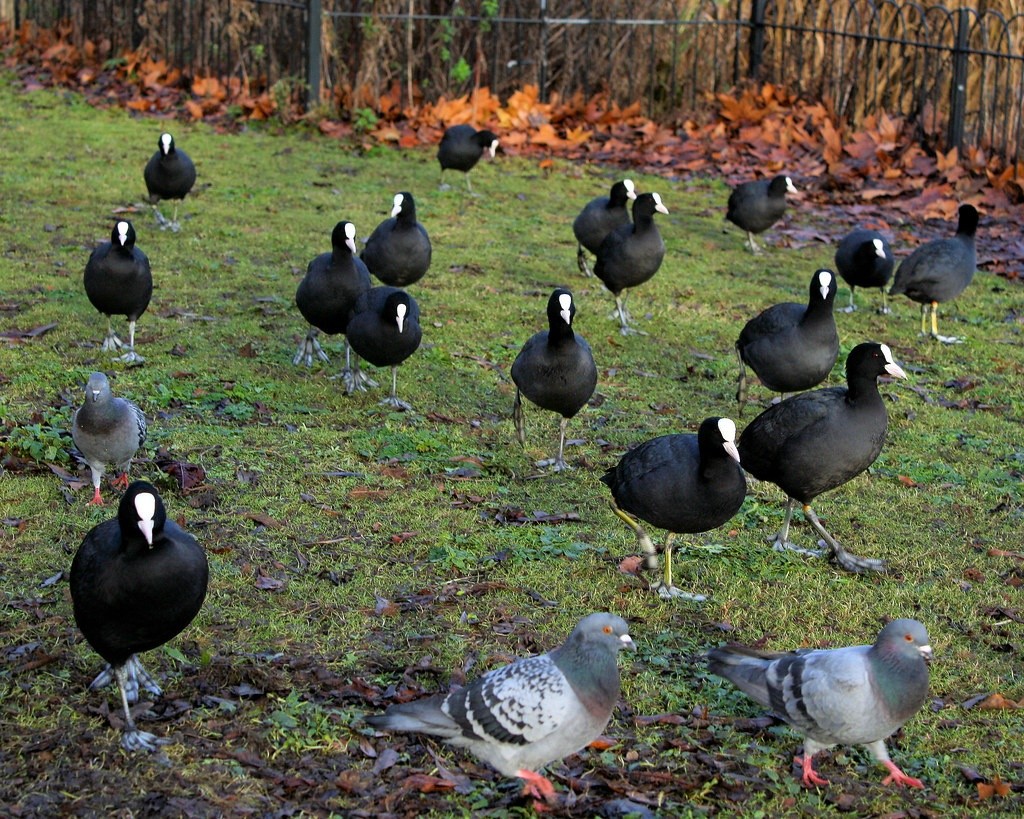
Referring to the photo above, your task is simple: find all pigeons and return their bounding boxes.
[361,608,638,803]
[71,370,148,509]
[706,618,939,792]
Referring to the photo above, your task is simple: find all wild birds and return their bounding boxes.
[888,198,981,343]
[143,131,198,233]
[598,415,749,604]
[593,191,670,338]
[834,229,896,316]
[726,174,800,254]
[69,481,210,753]
[733,268,840,420]
[738,341,920,575]
[436,122,500,198]
[292,189,432,411]
[82,220,155,369]
[510,289,601,472]
[571,177,638,278]
